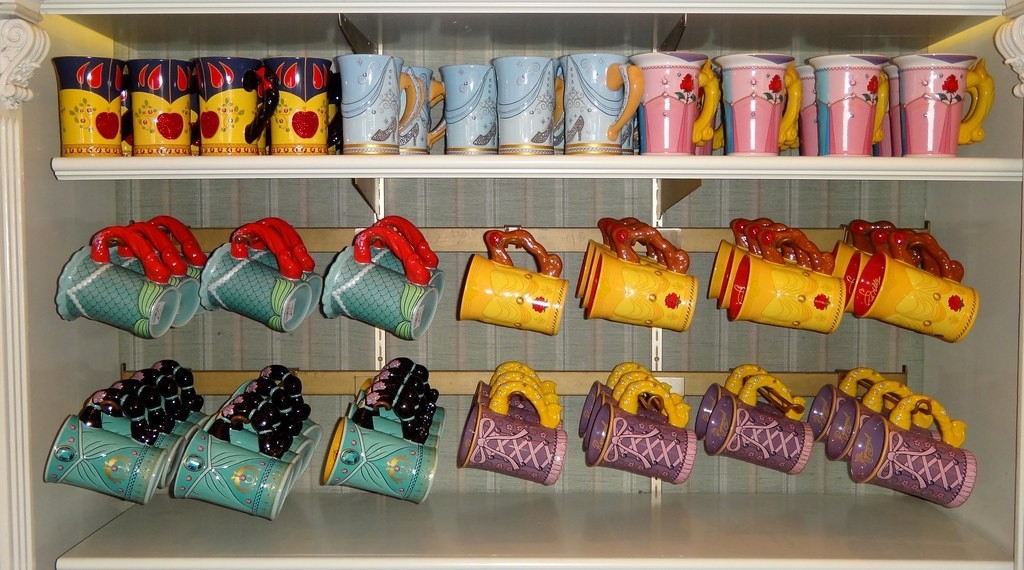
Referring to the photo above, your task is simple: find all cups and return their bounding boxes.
[694,365,813,475]
[574,218,698,335]
[707,218,846,336]
[56,214,209,340]
[458,228,567,336]
[198,216,325,333]
[833,219,980,344]
[320,215,444,342]
[578,362,698,485]
[43,358,213,505]
[44,49,996,159]
[322,357,446,505]
[808,367,978,510]
[174,364,322,521]
[456,360,568,486]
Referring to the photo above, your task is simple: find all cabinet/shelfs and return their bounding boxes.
[52,153,1024,570]
